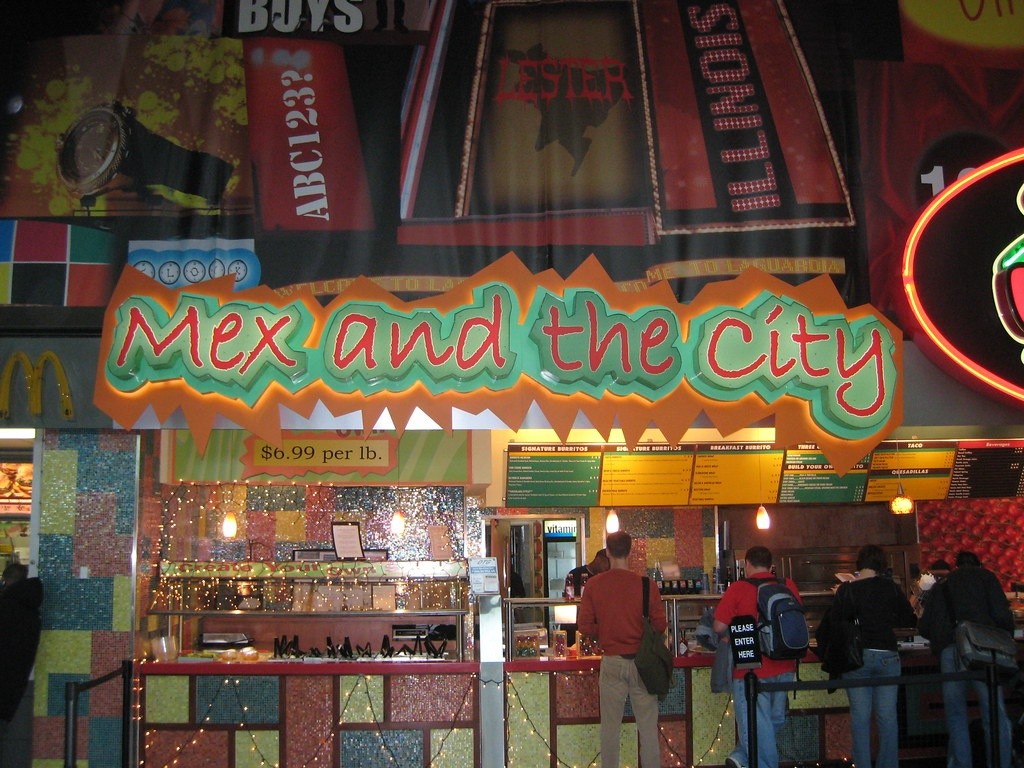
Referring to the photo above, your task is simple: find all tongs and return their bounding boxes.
[274,632,447,658]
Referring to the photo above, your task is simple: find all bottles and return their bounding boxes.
[564,574,575,600]
[581,574,589,599]
[679,630,688,657]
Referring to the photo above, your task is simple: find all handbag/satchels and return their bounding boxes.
[634,614,674,697]
[815,615,865,673]
[952,619,1020,675]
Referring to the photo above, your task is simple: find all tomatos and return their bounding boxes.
[917,497,1024,593]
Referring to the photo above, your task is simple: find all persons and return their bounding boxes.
[712,545,805,768]
[577,531,666,768]
[815,544,918,768]
[564,549,610,597]
[0,564,44,723]
[918,551,1024,768]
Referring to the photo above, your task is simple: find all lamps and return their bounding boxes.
[890,430,913,515]
[755,452,769,529]
[222,488,237,539]
[390,442,405,537]
[605,448,619,533]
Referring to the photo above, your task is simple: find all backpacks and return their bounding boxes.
[757,582,810,660]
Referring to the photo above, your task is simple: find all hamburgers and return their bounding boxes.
[0,461,34,497]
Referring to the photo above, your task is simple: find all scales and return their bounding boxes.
[200,632,254,648]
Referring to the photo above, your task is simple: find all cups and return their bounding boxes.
[700,573,711,593]
[718,584,725,594]
[152,637,178,659]
